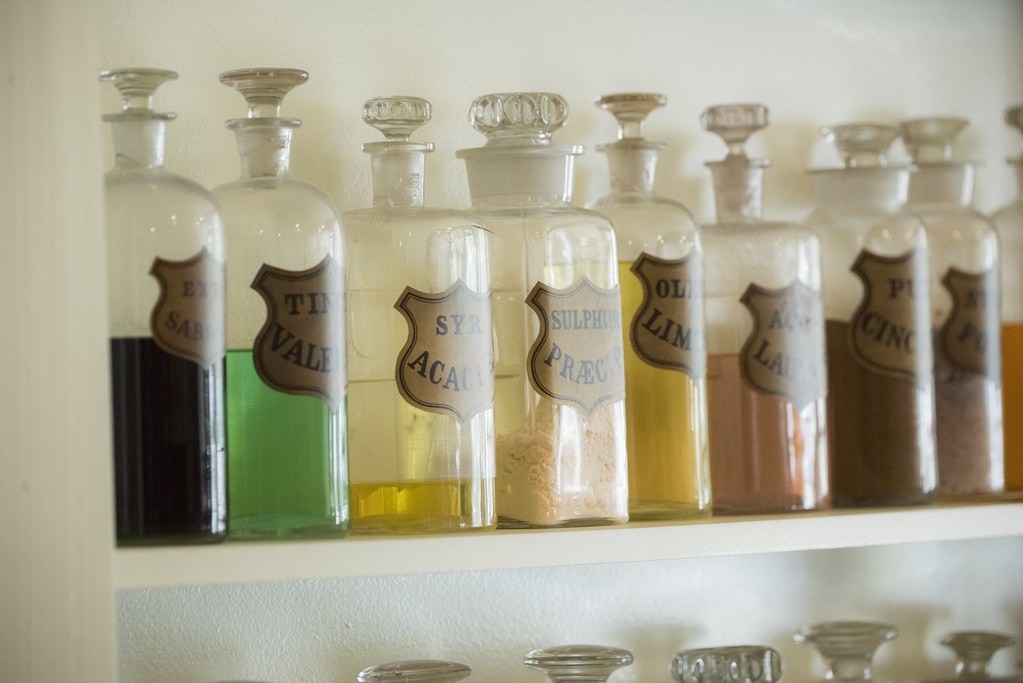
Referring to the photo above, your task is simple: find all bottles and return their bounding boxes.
[453,91,628,531]
[99,67,227,547]
[583,93,713,523]
[696,101,832,518]
[799,125,940,510]
[898,118,1006,503]
[208,68,350,542]
[355,622,1015,683]
[339,95,497,537]
[988,106,1022,502]
